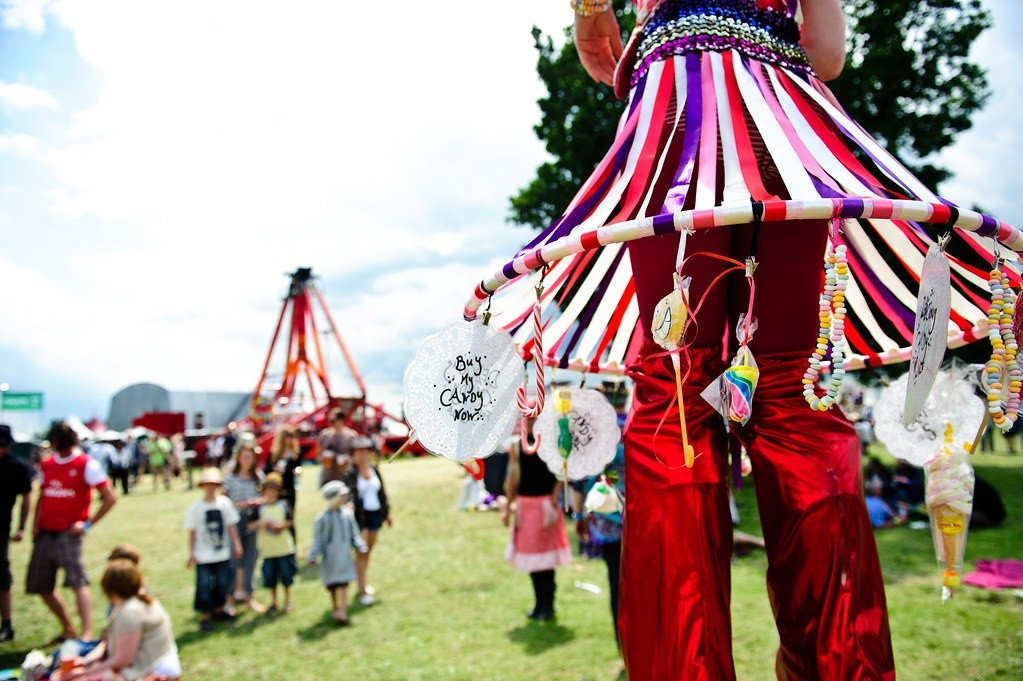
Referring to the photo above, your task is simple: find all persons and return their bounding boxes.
[26,424,118,643]
[79,433,235,495]
[401,0,1023,681]
[503,412,626,640]
[62,545,182,681]
[185,429,304,630]
[865,456,1006,530]
[0,424,34,641]
[308,408,393,622]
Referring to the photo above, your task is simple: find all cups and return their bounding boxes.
[59,660,74,681]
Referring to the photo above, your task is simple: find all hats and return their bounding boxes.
[264,472,283,489]
[197,467,222,486]
[321,481,351,500]
[350,436,373,451]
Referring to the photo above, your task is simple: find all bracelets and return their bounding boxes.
[570,0,612,16]
[85,522,91,529]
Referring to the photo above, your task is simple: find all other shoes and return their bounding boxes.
[74,636,96,656]
[52,634,66,646]
